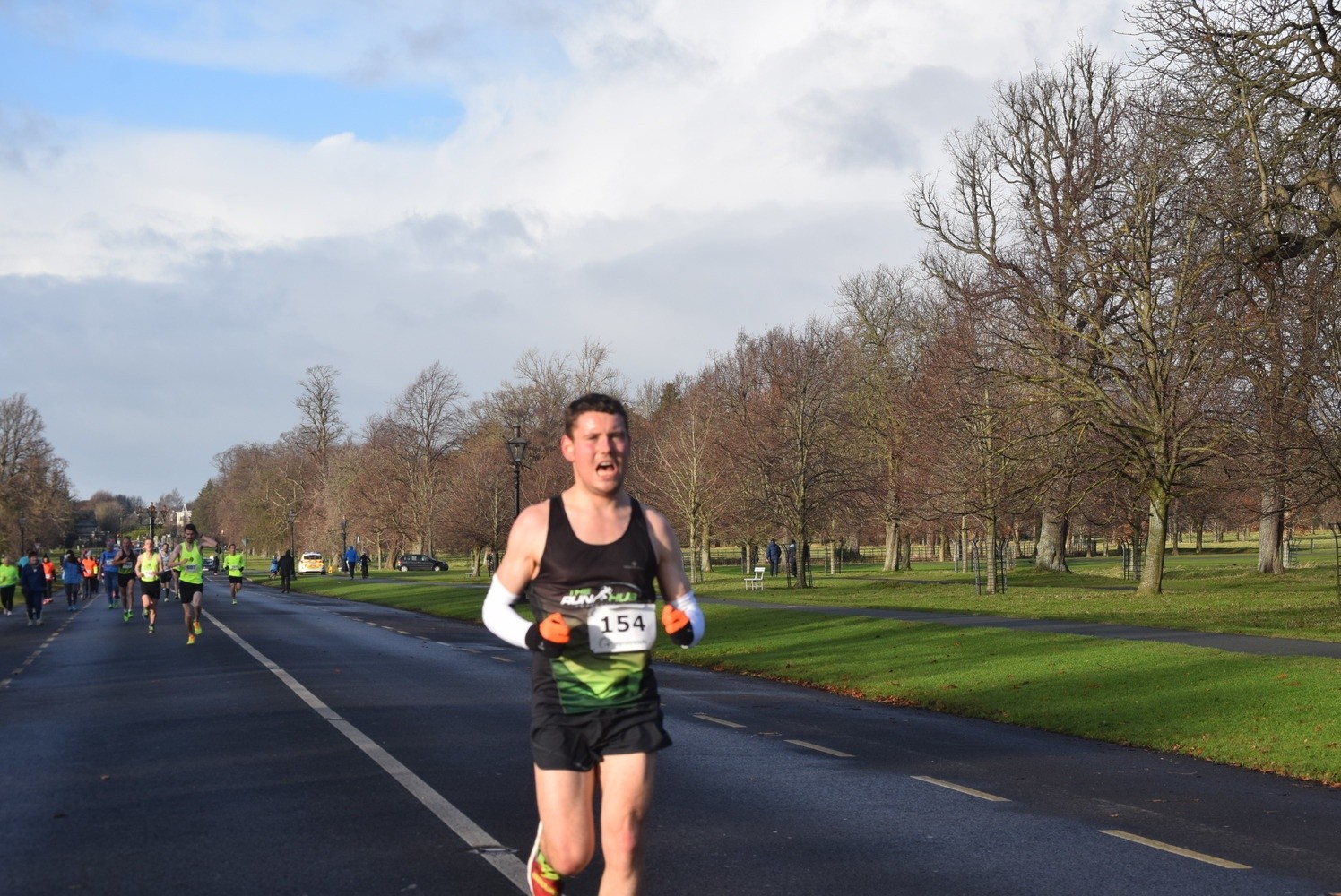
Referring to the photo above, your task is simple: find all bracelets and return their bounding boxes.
[200,534,203,539]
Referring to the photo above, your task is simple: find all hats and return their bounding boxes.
[67,550,74,556]
[43,553,48,558]
[85,551,92,556]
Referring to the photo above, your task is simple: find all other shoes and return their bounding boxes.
[67,606,76,610]
[187,633,195,645]
[235,589,237,593]
[148,624,154,634]
[141,609,149,619]
[123,612,129,623]
[127,610,132,617]
[115,601,118,608]
[233,599,237,604]
[164,598,169,602]
[108,604,112,608]
[4,608,12,616]
[193,620,202,635]
[36,619,43,626]
[42,598,50,605]
[174,595,178,598]
[27,620,33,625]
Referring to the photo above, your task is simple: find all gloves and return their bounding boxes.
[662,604,694,646]
[525,613,570,658]
[63,554,71,560]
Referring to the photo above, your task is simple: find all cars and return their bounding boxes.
[297,550,326,576]
[395,553,450,573]
[202,558,215,572]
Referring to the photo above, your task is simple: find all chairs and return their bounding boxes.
[744,567,765,591]
[465,567,475,577]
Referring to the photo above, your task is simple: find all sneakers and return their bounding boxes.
[526,821,566,896]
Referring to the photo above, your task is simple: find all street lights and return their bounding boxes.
[288,510,298,563]
[505,422,527,518]
[17,511,27,557]
[340,515,350,565]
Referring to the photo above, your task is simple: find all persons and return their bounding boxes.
[59,553,81,610]
[114,543,180,602]
[278,550,295,594]
[98,542,119,608]
[765,538,781,577]
[482,393,704,896]
[17,549,57,605]
[213,553,219,576]
[167,524,218,645]
[18,551,46,626]
[134,539,164,634]
[487,554,504,566]
[786,539,798,577]
[269,556,279,581]
[61,549,99,601]
[112,537,137,622]
[344,545,359,579]
[0,557,18,616]
[223,544,244,605]
[359,552,371,576]
